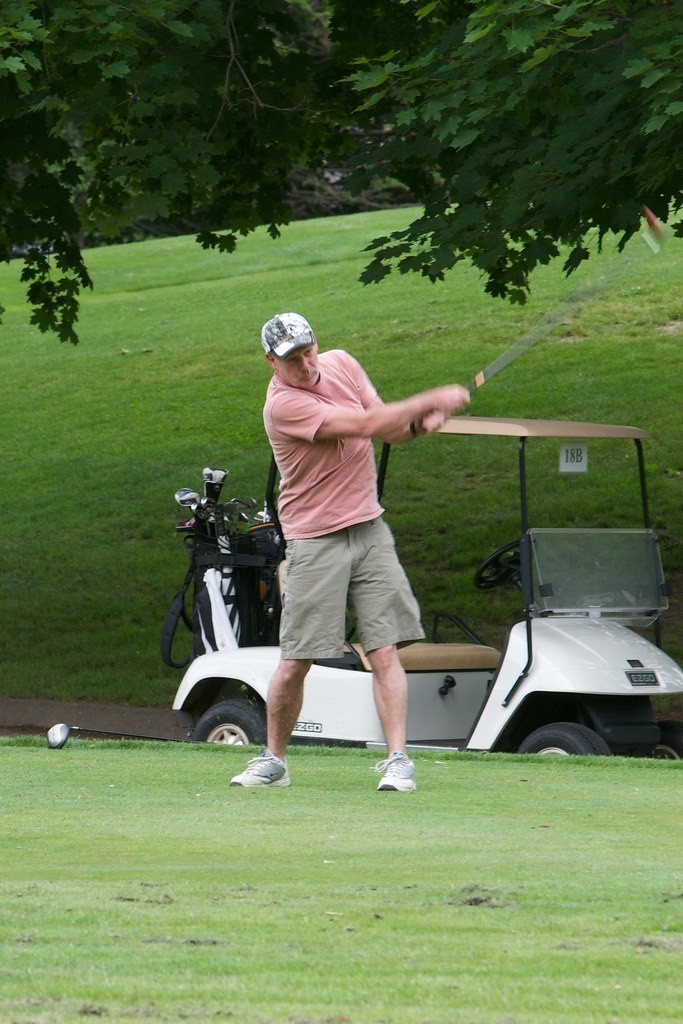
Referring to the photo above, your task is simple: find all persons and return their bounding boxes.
[229,313,471,793]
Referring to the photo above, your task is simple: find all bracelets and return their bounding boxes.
[410,420,418,435]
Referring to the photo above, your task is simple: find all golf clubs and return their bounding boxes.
[174,466,259,535]
[47,724,214,750]
[406,205,672,437]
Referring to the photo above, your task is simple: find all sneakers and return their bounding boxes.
[229,747,290,788]
[368,750,416,792]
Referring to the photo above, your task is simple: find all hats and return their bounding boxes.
[260,312,314,360]
